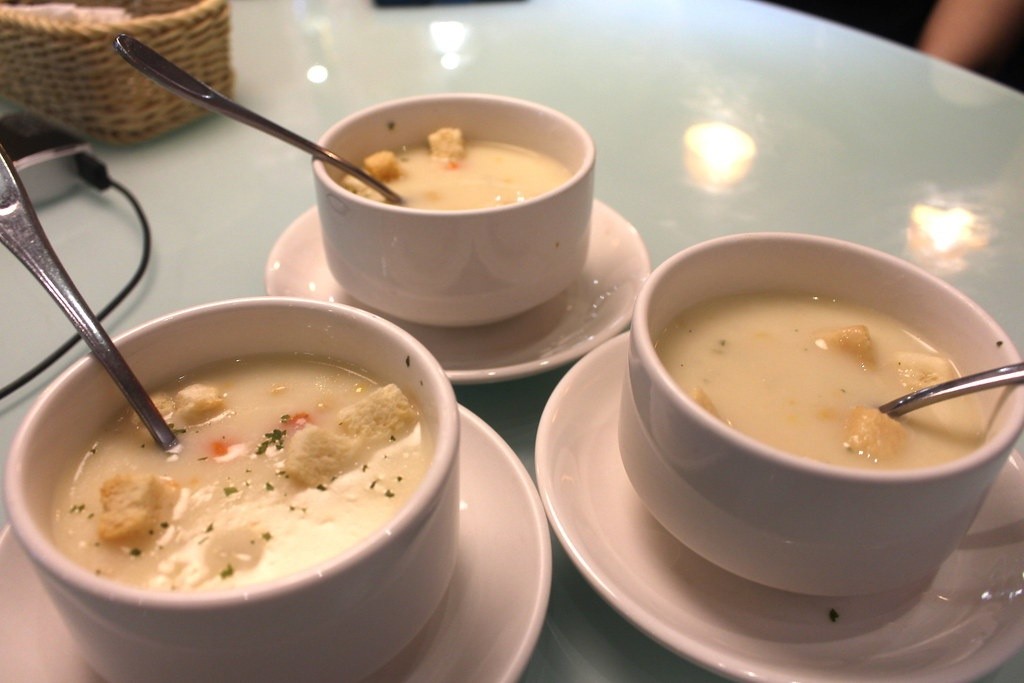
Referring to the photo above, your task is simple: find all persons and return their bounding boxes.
[770,0,1024,92]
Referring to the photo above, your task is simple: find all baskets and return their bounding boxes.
[0,0,234,144]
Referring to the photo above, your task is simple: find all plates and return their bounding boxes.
[534,328,1021,682]
[264,193,649,386]
[0,401,551,680]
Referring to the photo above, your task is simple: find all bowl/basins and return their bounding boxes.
[313,93,595,326]
[2,299,460,682]
[618,232,1023,599]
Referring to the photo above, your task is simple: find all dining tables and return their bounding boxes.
[0,0,1024,682]
